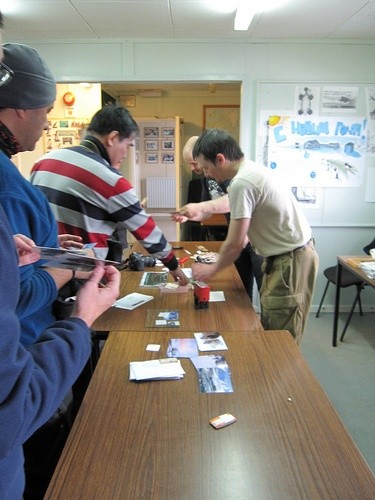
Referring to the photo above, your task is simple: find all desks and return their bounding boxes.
[44,331,375,500]
[200,214,227,240]
[333,254,375,347]
[89,241,264,374]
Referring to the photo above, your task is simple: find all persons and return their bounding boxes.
[0,12,120,500]
[31,105,188,287]
[190,128,319,347]
[0,44,73,459]
[172,136,264,305]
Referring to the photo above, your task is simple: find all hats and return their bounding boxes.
[0,43,56,110]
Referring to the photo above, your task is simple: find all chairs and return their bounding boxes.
[314,237,375,341]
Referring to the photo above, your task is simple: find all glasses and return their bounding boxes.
[0,61,15,86]
